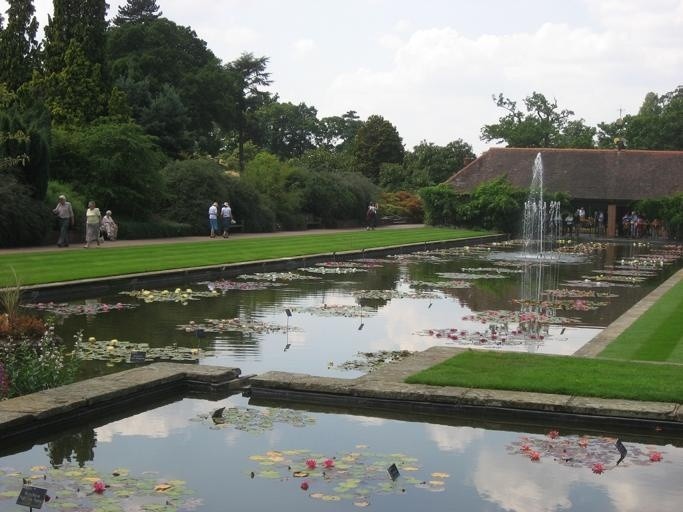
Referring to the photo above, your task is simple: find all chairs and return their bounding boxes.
[548,215,664,238]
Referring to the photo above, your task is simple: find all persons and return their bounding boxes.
[83,200,101,248]
[52,195,74,248]
[559,205,649,238]
[366,202,379,230]
[220,202,233,238]
[100,210,117,240]
[208,202,220,238]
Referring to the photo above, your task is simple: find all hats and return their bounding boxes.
[224,202,229,206]
[213,202,218,206]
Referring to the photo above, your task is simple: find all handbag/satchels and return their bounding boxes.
[231,219,236,224]
[98,237,104,243]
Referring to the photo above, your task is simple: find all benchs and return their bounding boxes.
[301,212,322,229]
[217,218,244,234]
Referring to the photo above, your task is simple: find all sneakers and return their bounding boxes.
[96,245,100,247]
[84,245,89,248]
